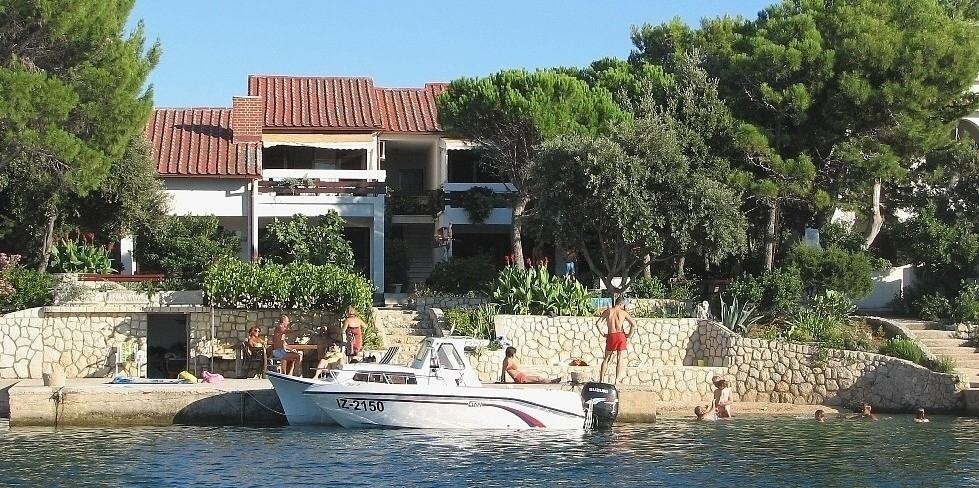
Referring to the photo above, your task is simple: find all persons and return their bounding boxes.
[913,407,929,423]
[498,347,561,384]
[562,249,578,275]
[815,410,825,422]
[712,376,734,417]
[695,399,716,420]
[248,304,367,380]
[596,297,635,384]
[861,403,877,420]
[716,380,729,418]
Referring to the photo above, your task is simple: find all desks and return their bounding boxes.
[283,344,319,377]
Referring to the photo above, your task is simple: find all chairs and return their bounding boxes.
[240,338,288,380]
[310,342,344,383]
